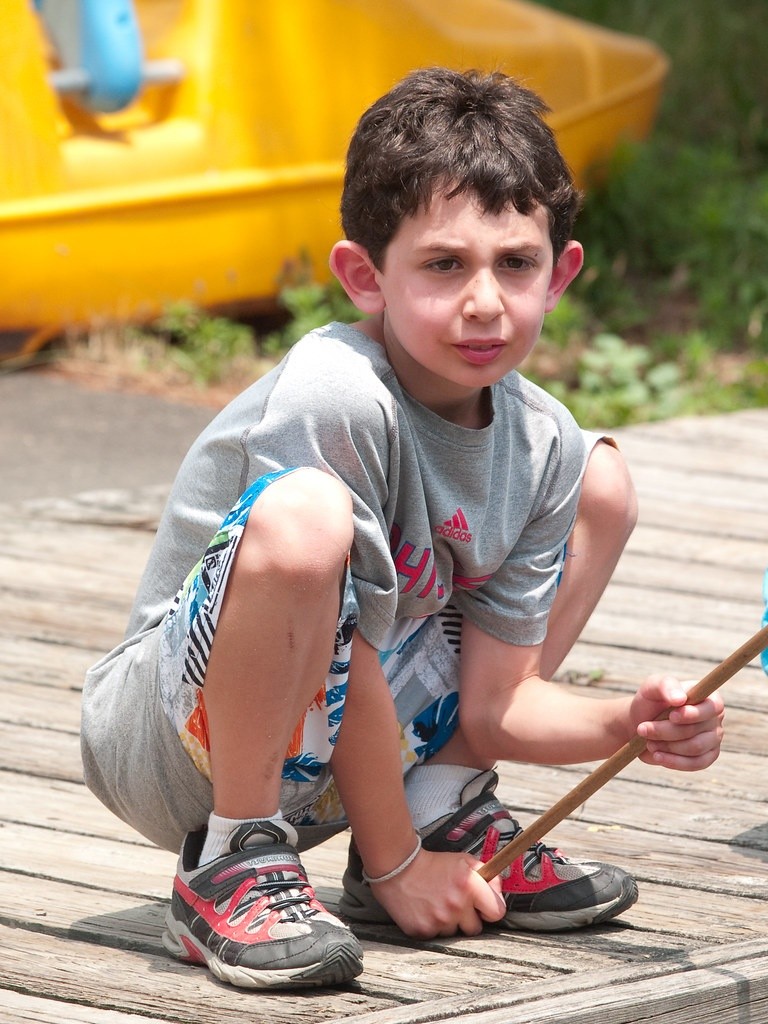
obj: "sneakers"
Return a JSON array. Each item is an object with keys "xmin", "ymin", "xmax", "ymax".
[
  {"xmin": 160, "ymin": 825, "xmax": 364, "ymax": 992},
  {"xmin": 339, "ymin": 764, "xmax": 638, "ymax": 932}
]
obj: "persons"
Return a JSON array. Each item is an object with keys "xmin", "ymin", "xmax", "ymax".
[{"xmin": 80, "ymin": 68, "xmax": 724, "ymax": 989}]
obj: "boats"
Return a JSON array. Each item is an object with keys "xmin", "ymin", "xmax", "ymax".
[{"xmin": 0, "ymin": 1, "xmax": 674, "ymax": 335}]
